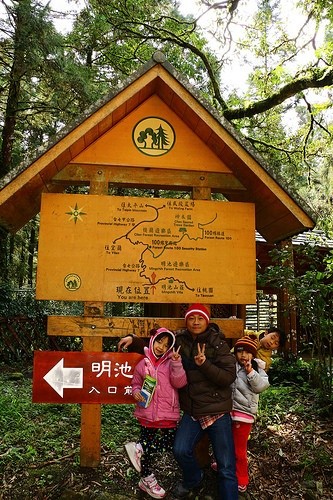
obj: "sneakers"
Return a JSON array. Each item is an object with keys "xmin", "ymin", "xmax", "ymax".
[
  {"xmin": 138, "ymin": 472, "xmax": 166, "ymax": 499},
  {"xmin": 124, "ymin": 441, "xmax": 143, "ymax": 473}
]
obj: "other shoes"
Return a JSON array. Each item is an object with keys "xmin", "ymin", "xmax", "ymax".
[
  {"xmin": 211, "ymin": 462, "xmax": 217, "ymax": 471},
  {"xmin": 175, "ymin": 470, "xmax": 204, "ymax": 497},
  {"xmin": 238, "ymin": 484, "xmax": 246, "ymax": 492}
]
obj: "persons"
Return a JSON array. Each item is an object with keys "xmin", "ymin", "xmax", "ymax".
[
  {"xmin": 117, "ymin": 304, "xmax": 237, "ymax": 500},
  {"xmin": 229, "ymin": 315, "xmax": 286, "ymax": 372},
  {"xmin": 211, "ymin": 335, "xmax": 270, "ymax": 492},
  {"xmin": 124, "ymin": 328, "xmax": 187, "ymax": 499}
]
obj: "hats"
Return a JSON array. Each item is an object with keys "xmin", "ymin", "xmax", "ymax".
[
  {"xmin": 185, "ymin": 304, "xmax": 210, "ymax": 324},
  {"xmin": 234, "ymin": 334, "xmax": 257, "ymax": 356}
]
